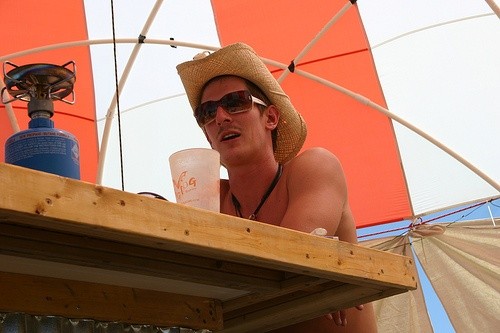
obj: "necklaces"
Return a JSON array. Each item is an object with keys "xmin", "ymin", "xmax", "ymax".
[{"xmin": 222, "ymin": 163, "xmax": 283, "ymax": 220}]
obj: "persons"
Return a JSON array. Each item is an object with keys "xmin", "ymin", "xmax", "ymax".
[{"xmin": 176, "ymin": 41, "xmax": 378, "ymax": 333}]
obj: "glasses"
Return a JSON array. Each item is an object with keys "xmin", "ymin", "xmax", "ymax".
[{"xmin": 193, "ymin": 90, "xmax": 269, "ymax": 128}]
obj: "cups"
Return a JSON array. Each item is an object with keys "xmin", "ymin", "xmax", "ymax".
[{"xmin": 169, "ymin": 148, "xmax": 221, "ymax": 213}]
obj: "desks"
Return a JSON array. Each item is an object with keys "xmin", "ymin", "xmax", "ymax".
[{"xmin": 0, "ymin": 162, "xmax": 418, "ymax": 333}]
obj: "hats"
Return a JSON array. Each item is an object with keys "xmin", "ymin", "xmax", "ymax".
[{"xmin": 176, "ymin": 42, "xmax": 307, "ymax": 163}]
